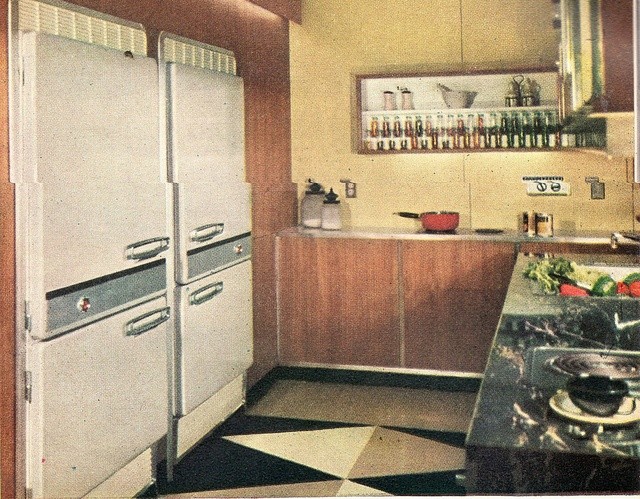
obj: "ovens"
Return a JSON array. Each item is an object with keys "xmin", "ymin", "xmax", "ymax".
[
  {"xmin": 8, "ymin": 0, "xmax": 169, "ymax": 498},
  {"xmin": 158, "ymin": 25, "xmax": 254, "ymax": 467}
]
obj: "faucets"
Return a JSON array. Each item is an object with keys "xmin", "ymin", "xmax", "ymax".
[{"xmin": 611, "ymin": 230, "xmax": 639, "ymax": 249}]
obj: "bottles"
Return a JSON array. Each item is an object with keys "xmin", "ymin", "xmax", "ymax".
[
  {"xmin": 404, "ymin": 116, "xmax": 413, "ymax": 152},
  {"xmin": 381, "ymin": 114, "xmax": 392, "ymax": 153},
  {"xmin": 468, "ymin": 113, "xmax": 477, "ymax": 148},
  {"xmin": 559, "ymin": 127, "xmax": 569, "ymax": 148},
  {"xmin": 567, "ymin": 124, "xmax": 577, "ymax": 148},
  {"xmin": 455, "ymin": 113, "xmax": 467, "ymax": 148},
  {"xmin": 546, "ymin": 111, "xmax": 557, "ymax": 148},
  {"xmin": 414, "ymin": 115, "xmax": 423, "ymax": 153},
  {"xmin": 391, "ymin": 114, "xmax": 405, "ymax": 153},
  {"xmin": 511, "ymin": 112, "xmax": 522, "ymax": 150},
  {"xmin": 424, "ymin": 115, "xmax": 435, "ymax": 150},
  {"xmin": 501, "ymin": 111, "xmax": 510, "ymax": 150},
  {"xmin": 435, "ymin": 114, "xmax": 446, "ymax": 149},
  {"xmin": 488, "ymin": 112, "xmax": 499, "ymax": 150},
  {"xmin": 477, "ymin": 113, "xmax": 488, "ymax": 148},
  {"xmin": 445, "ymin": 112, "xmax": 456, "ymax": 150},
  {"xmin": 368, "ymin": 115, "xmax": 382, "ymax": 149},
  {"xmin": 522, "ymin": 112, "xmax": 534, "ymax": 148},
  {"xmin": 535, "ymin": 111, "xmax": 546, "ymax": 149}
]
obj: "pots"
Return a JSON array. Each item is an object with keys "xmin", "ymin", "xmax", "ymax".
[{"xmin": 393, "ymin": 209, "xmax": 460, "ymax": 233}]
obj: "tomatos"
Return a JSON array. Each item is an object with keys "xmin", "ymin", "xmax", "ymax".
[
  {"xmin": 630, "ymin": 281, "xmax": 640, "ymax": 297},
  {"xmin": 615, "ymin": 281, "xmax": 629, "ymax": 296}
]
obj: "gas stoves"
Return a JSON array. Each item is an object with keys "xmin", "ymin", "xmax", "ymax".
[
  {"xmin": 414, "ymin": 227, "xmax": 518, "ymax": 239},
  {"xmin": 465, "ymin": 314, "xmax": 639, "ymax": 459}
]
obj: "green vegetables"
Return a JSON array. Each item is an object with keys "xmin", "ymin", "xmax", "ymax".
[{"xmin": 522, "ymin": 259, "xmax": 559, "ymax": 291}]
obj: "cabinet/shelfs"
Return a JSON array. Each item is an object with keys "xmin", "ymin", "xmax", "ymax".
[
  {"xmin": 274, "ymin": 227, "xmax": 640, "ymax": 382},
  {"xmin": 350, "ymin": 62, "xmax": 609, "ymax": 154},
  {"xmin": 558, "ymin": 1, "xmax": 639, "ymax": 117}
]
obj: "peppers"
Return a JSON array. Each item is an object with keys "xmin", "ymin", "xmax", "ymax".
[{"xmin": 591, "ymin": 275, "xmax": 617, "ymax": 296}]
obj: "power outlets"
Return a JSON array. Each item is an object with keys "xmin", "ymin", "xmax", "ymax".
[{"xmin": 345, "ymin": 181, "xmax": 356, "ymax": 198}]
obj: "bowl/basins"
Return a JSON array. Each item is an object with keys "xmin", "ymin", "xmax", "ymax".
[
  {"xmin": 442, "ymin": 88, "xmax": 478, "ymax": 109},
  {"xmin": 565, "ymin": 374, "xmax": 630, "ymax": 418}
]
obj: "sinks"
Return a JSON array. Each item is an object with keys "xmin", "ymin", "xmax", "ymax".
[{"xmin": 567, "ymin": 297, "xmax": 639, "ymax": 352}]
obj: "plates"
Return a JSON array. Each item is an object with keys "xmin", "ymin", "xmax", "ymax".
[{"xmin": 549, "ymin": 392, "xmax": 640, "ymax": 428}]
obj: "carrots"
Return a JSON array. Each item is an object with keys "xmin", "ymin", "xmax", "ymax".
[{"xmin": 560, "ymin": 282, "xmax": 588, "ymax": 297}]
